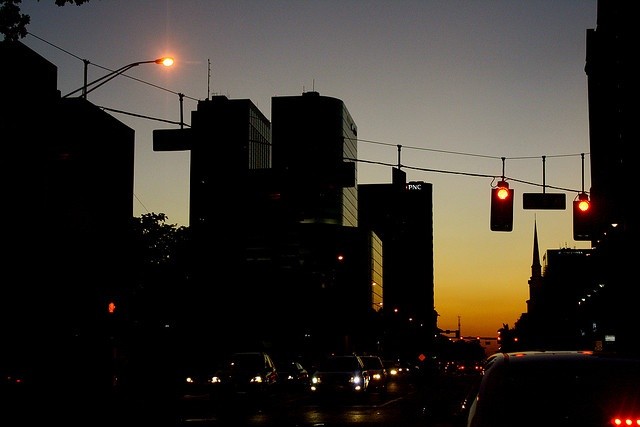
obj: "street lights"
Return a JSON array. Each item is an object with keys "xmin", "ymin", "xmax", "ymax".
[{"xmin": 61, "ymin": 58, "xmax": 174, "ymax": 99}]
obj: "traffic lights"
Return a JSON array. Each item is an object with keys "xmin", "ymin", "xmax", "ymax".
[
  {"xmin": 573, "ymin": 201, "xmax": 593, "ymax": 241},
  {"xmin": 491, "ymin": 189, "xmax": 513, "ymax": 231}
]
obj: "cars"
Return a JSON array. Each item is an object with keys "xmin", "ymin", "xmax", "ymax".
[
  {"xmin": 359, "ymin": 356, "xmax": 388, "ymax": 389},
  {"xmin": 385, "ymin": 357, "xmax": 419, "ymax": 378},
  {"xmin": 212, "ymin": 353, "xmax": 279, "ymax": 384},
  {"xmin": 467, "ymin": 351, "xmax": 611, "ymax": 427},
  {"xmin": 311, "ymin": 355, "xmax": 370, "ymax": 392}
]
